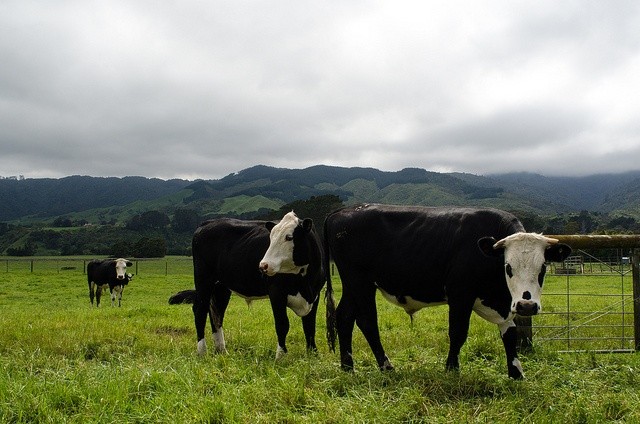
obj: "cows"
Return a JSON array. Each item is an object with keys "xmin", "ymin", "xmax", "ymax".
[
  {"xmin": 104, "ymin": 273, "xmax": 134, "ymax": 295},
  {"xmin": 322, "ymin": 203, "xmax": 573, "ymax": 380},
  {"xmin": 87, "ymin": 258, "xmax": 133, "ymax": 308},
  {"xmin": 168, "ymin": 290, "xmax": 196, "ymax": 305},
  {"xmin": 191, "ymin": 210, "xmax": 327, "ymax": 360}
]
